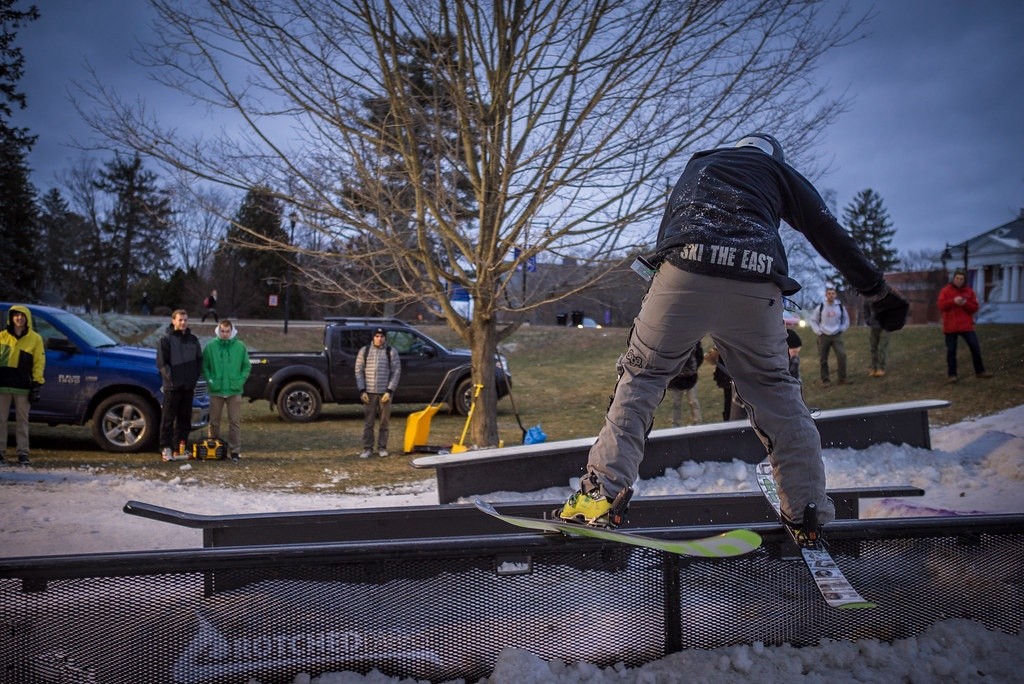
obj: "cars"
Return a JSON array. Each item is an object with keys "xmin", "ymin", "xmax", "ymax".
[
  {"xmin": 567, "ymin": 317, "xmax": 602, "ymax": 329},
  {"xmin": 783, "ymin": 309, "xmax": 806, "ymax": 327}
]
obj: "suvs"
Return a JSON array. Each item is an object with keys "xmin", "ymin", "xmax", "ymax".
[{"xmin": 0, "ymin": 301, "xmax": 212, "ymax": 454}]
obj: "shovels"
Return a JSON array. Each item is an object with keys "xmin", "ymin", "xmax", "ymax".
[
  {"xmin": 495, "ymin": 347, "xmax": 527, "ymax": 445},
  {"xmin": 450, "ymin": 385, "xmax": 485, "ymax": 454}
]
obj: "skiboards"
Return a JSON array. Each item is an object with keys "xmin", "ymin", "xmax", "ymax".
[{"xmin": 472, "ymin": 461, "xmax": 880, "ymax": 610}]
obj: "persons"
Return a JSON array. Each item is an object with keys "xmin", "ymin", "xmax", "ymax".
[
  {"xmin": 668, "ymin": 288, "xmax": 894, "ymax": 425},
  {"xmin": 556, "ymin": 132, "xmax": 915, "ymax": 547},
  {"xmin": 937, "ymin": 271, "xmax": 995, "ymax": 382},
  {"xmin": 203, "ymin": 319, "xmax": 250, "ymax": 462},
  {"xmin": 356, "ymin": 327, "xmax": 400, "ymax": 460},
  {"xmin": 0, "ymin": 306, "xmax": 46, "ymax": 467},
  {"xmin": 202, "ymin": 289, "xmax": 220, "ymax": 325},
  {"xmin": 158, "ymin": 310, "xmax": 202, "ymax": 462}
]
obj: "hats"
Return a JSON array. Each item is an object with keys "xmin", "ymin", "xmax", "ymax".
[
  {"xmin": 372, "ymin": 326, "xmax": 387, "ymax": 338},
  {"xmin": 786, "ymin": 329, "xmax": 801, "ymax": 349}
]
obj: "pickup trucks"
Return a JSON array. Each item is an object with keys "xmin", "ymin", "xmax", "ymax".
[{"xmin": 241, "ymin": 317, "xmax": 512, "ymax": 425}]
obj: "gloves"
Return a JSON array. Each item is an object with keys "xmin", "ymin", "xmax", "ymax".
[{"xmin": 870, "ymin": 289, "xmax": 908, "ymax": 331}]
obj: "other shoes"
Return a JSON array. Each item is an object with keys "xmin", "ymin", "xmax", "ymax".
[
  {"xmin": 977, "ymin": 372, "xmax": 992, "ymax": 378},
  {"xmin": 839, "ymin": 378, "xmax": 853, "ymax": 385},
  {"xmin": 949, "ymin": 376, "xmax": 958, "ymax": 383},
  {"xmin": 868, "ymin": 370, "xmax": 875, "ymax": 376},
  {"xmin": 875, "ymin": 369, "xmax": 885, "ymax": 376},
  {"xmin": 824, "ymin": 381, "xmax": 832, "ymax": 387},
  {"xmin": 19, "ymin": 455, "xmax": 31, "ymax": 464},
  {"xmin": 230, "ymin": 452, "xmax": 240, "ymax": 460}
]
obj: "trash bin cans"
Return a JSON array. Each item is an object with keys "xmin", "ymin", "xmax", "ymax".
[
  {"xmin": 556, "ymin": 313, "xmax": 568, "ymax": 326},
  {"xmin": 571, "ymin": 310, "xmax": 584, "ymax": 326}
]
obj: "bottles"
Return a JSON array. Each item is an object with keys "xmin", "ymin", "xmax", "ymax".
[{"xmin": 179, "ymin": 441, "xmax": 185, "ymax": 455}]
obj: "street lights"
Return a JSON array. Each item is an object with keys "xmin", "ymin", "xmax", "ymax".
[
  {"xmin": 230, "ymin": 251, "xmax": 248, "ymax": 317},
  {"xmin": 283, "ymin": 211, "xmax": 298, "ymax": 334},
  {"xmin": 941, "ymin": 240, "xmax": 969, "ymax": 274},
  {"xmin": 521, "ymin": 220, "xmax": 553, "ymax": 323}
]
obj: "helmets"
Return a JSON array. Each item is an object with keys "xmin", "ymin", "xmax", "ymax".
[{"xmin": 734, "ymin": 132, "xmax": 785, "ymax": 162}]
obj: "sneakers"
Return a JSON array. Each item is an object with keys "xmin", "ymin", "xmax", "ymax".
[
  {"xmin": 162, "ymin": 448, "xmax": 174, "ymax": 462},
  {"xmin": 559, "ymin": 488, "xmax": 620, "ymax": 527},
  {"xmin": 793, "ymin": 530, "xmax": 820, "ymax": 546},
  {"xmin": 379, "ymin": 449, "xmax": 388, "ymax": 457},
  {"xmin": 359, "ymin": 450, "xmax": 372, "ymax": 459}
]
